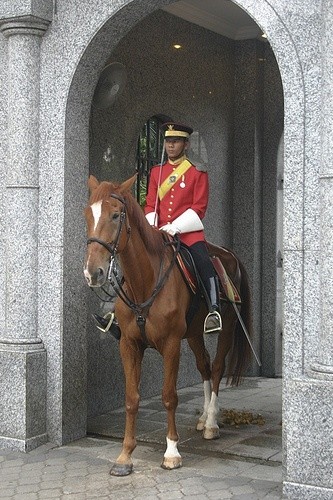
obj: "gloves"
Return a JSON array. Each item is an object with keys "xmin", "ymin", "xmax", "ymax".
[
  {"xmin": 159, "ymin": 208, "xmax": 205, "ymax": 237},
  {"xmin": 145, "ymin": 212, "xmax": 159, "ymax": 230}
]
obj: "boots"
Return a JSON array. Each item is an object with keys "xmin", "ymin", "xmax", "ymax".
[
  {"xmin": 203, "ymin": 275, "xmax": 222, "ymax": 334},
  {"xmin": 91, "ymin": 312, "xmax": 121, "ymax": 340}
]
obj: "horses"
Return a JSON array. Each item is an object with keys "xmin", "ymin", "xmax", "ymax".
[{"xmin": 83, "ymin": 172, "xmax": 253, "ymax": 478}]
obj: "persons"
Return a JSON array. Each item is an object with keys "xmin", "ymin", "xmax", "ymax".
[{"xmin": 91, "ymin": 122, "xmax": 220, "ymax": 340}]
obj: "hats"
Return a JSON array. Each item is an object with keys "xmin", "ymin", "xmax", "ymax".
[{"xmin": 162, "ymin": 123, "xmax": 193, "ymax": 142}]
